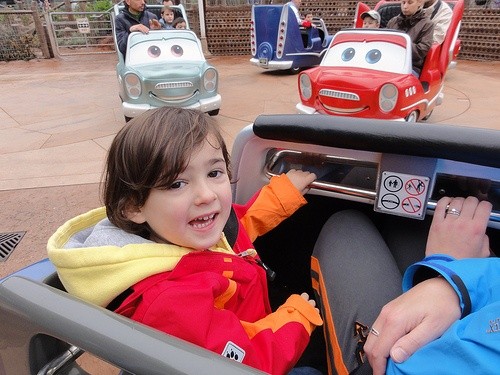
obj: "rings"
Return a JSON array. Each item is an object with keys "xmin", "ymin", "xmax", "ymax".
[
  {"xmin": 447, "ymin": 208, "xmax": 460, "ymax": 217},
  {"xmin": 371, "ymin": 328, "xmax": 380, "ymax": 336}
]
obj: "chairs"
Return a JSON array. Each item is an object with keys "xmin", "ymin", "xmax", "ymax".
[
  {"xmin": 0, "ymin": 277, "xmax": 268, "ymax": 375},
  {"xmin": 119, "ymin": 7, "xmax": 183, "ymax": 20},
  {"xmin": 299, "ymin": 20, "xmax": 325, "ymax": 48},
  {"xmin": 376, "ymin": 2, "xmax": 454, "ymax": 28}
]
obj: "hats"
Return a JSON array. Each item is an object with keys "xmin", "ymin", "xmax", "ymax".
[{"xmin": 361, "ymin": 9, "xmax": 381, "ymax": 24}]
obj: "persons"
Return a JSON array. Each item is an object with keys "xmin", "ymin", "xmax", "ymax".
[
  {"xmin": 114, "ymin": 1, "xmax": 188, "ymax": 56},
  {"xmin": 47, "ymin": 108, "xmax": 326, "ymax": 375},
  {"xmin": 361, "ymin": 1, "xmax": 453, "ymax": 93},
  {"xmin": 288, "ymin": 0, "xmax": 324, "ymax": 49},
  {"xmin": 310, "ymin": 195, "xmax": 500, "ymax": 375}
]
